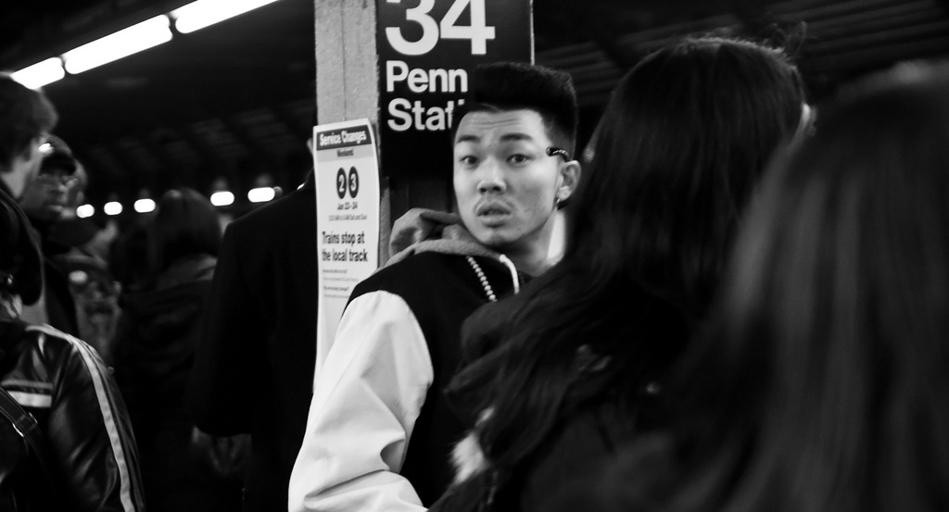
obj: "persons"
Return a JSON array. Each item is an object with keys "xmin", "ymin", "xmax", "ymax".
[
  {"xmin": 0, "ymin": 71, "xmax": 317, "ymax": 512},
  {"xmin": 537, "ymin": 62, "xmax": 946, "ymax": 511},
  {"xmin": 283, "ymin": 52, "xmax": 587, "ymax": 511},
  {"xmin": 426, "ymin": 39, "xmax": 819, "ymax": 509}
]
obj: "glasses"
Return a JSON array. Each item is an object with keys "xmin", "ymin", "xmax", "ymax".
[{"xmin": 30, "ymin": 175, "xmax": 82, "ymax": 190}]
